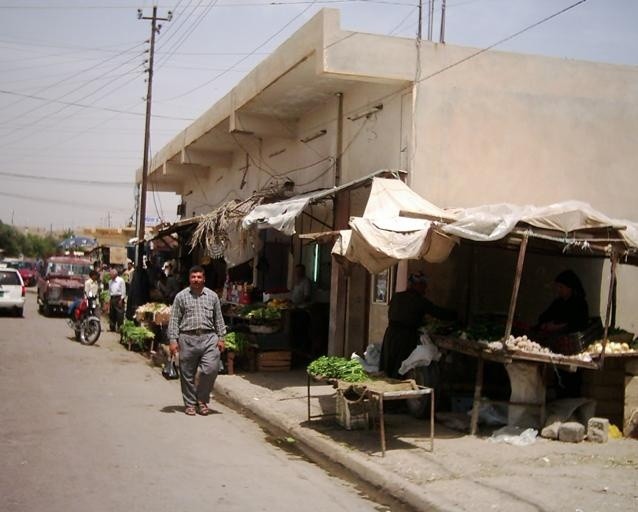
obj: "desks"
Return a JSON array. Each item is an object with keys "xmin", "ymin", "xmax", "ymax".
[{"xmin": 306, "ymin": 367, "xmax": 436, "ymax": 456}]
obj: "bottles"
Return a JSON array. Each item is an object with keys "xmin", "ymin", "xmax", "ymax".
[{"xmin": 227, "ymin": 282, "xmax": 247, "ymax": 304}]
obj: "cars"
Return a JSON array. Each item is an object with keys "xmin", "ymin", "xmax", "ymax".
[{"xmin": 0, "ymin": 256, "xmax": 92, "ymax": 317}]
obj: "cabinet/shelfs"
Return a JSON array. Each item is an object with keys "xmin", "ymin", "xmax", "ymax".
[
  {"xmin": 120, "ymin": 332, "xmax": 154, "ymax": 353},
  {"xmin": 223, "ymin": 312, "xmax": 284, "ymax": 375},
  {"xmin": 583, "ymin": 350, "xmax": 638, "ymax": 436},
  {"xmin": 430, "ymin": 333, "xmax": 600, "ymax": 435}
]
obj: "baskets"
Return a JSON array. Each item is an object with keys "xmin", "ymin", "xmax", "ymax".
[{"xmin": 257, "ymin": 350, "xmax": 293, "ymax": 372}]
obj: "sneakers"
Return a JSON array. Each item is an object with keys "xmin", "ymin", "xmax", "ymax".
[
  {"xmin": 185, "ymin": 405, "xmax": 198, "ymax": 416},
  {"xmin": 199, "ymin": 399, "xmax": 209, "ymax": 415}
]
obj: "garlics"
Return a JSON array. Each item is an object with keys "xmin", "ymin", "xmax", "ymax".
[{"xmin": 588, "ymin": 339, "xmax": 633, "ymax": 353}]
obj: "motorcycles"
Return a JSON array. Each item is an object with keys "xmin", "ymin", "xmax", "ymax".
[{"xmin": 66, "ymin": 287, "xmax": 102, "ymax": 345}]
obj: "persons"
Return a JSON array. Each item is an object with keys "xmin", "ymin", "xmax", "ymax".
[
  {"xmin": 376, "ymin": 269, "xmax": 463, "ymax": 415},
  {"xmin": 165, "ymin": 264, "xmax": 229, "ymax": 416},
  {"xmin": 535, "ymin": 269, "xmax": 589, "ymax": 422},
  {"xmin": 95, "ymin": 260, "xmax": 181, "ymax": 305},
  {"xmin": 286, "ymin": 263, "xmax": 313, "ymax": 367},
  {"xmin": 107, "ymin": 269, "xmax": 127, "ymax": 332},
  {"xmin": 85, "ymin": 269, "xmax": 99, "ymax": 316}
]
